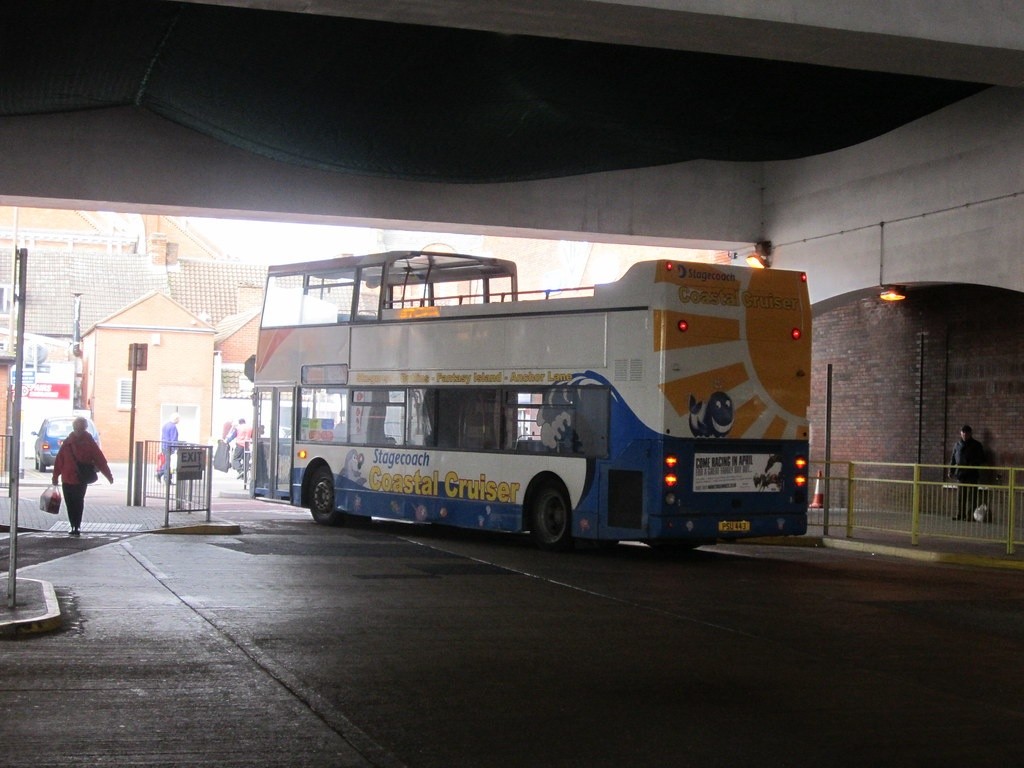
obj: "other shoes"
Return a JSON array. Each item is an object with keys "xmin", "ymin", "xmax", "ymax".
[
  {"xmin": 237, "ymin": 471, "xmax": 245, "ymax": 480},
  {"xmin": 953, "ymin": 515, "xmax": 975, "ymax": 521},
  {"xmin": 169, "ymin": 481, "xmax": 174, "ymax": 485},
  {"xmin": 155, "ymin": 473, "xmax": 161, "ymax": 483}
]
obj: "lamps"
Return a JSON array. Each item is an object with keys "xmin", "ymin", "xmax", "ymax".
[
  {"xmin": 880, "ymin": 290, "xmax": 906, "ymax": 299},
  {"xmin": 747, "ymin": 255, "xmax": 769, "ymax": 269}
]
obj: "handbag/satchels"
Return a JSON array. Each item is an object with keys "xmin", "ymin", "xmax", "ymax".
[
  {"xmin": 76, "ymin": 463, "xmax": 98, "ymax": 484},
  {"xmin": 39, "ymin": 483, "xmax": 62, "ymax": 514},
  {"xmin": 157, "ymin": 452, "xmax": 165, "ymax": 472}
]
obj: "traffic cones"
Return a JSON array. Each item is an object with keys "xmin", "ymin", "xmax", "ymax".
[{"xmin": 808, "ymin": 470, "xmax": 830, "ymax": 509}]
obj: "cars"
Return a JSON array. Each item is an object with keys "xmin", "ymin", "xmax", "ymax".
[{"xmin": 30, "ymin": 415, "xmax": 102, "ymax": 473}]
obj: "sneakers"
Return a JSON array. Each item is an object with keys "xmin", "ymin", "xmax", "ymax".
[{"xmin": 68, "ymin": 526, "xmax": 80, "ymax": 537}]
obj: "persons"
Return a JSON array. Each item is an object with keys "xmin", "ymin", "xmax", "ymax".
[
  {"xmin": 226, "ymin": 418, "xmax": 253, "ymax": 480},
  {"xmin": 948, "ymin": 426, "xmax": 989, "ymax": 521},
  {"xmin": 155, "ymin": 413, "xmax": 179, "ymax": 485},
  {"xmin": 52, "ymin": 417, "xmax": 113, "ymax": 535}
]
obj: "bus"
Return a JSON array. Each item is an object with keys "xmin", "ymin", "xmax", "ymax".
[{"xmin": 246, "ymin": 249, "xmax": 815, "ymax": 556}]
